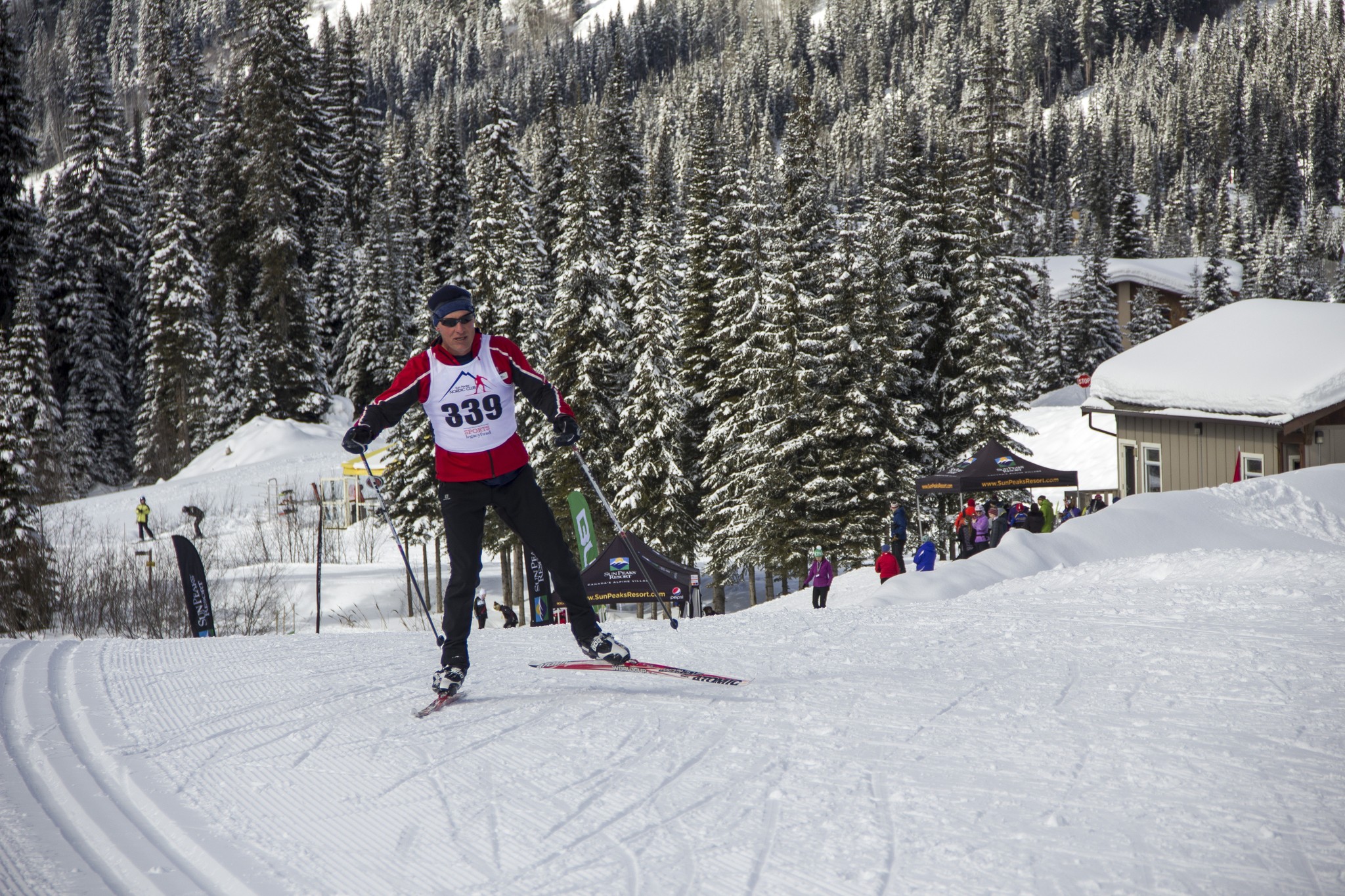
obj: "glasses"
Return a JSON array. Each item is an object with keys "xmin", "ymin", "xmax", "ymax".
[
  {"xmin": 433, "ymin": 305, "xmax": 475, "ymax": 327},
  {"xmin": 975, "ymin": 510, "xmax": 983, "ymax": 513}
]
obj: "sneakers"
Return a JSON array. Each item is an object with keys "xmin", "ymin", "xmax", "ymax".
[
  {"xmin": 580, "ymin": 633, "xmax": 631, "ymax": 665},
  {"xmin": 433, "ymin": 663, "xmax": 467, "ymax": 696}
]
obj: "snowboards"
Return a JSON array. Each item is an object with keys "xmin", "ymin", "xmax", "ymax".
[{"xmin": 171, "ymin": 534, "xmax": 216, "ymax": 640}]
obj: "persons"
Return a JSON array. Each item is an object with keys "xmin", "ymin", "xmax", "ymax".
[
  {"xmin": 494, "ymin": 601, "xmax": 519, "ymax": 628},
  {"xmin": 875, "ymin": 496, "xmax": 1121, "ymax": 585},
  {"xmin": 703, "ymin": 606, "xmax": 720, "ymax": 617},
  {"xmin": 135, "ymin": 496, "xmax": 155, "ymax": 542},
  {"xmin": 341, "ymin": 286, "xmax": 631, "ymax": 695},
  {"xmin": 182, "ymin": 505, "xmax": 205, "ymax": 539},
  {"xmin": 802, "ymin": 545, "xmax": 833, "ymax": 609},
  {"xmin": 473, "ymin": 588, "xmax": 488, "ymax": 629}
]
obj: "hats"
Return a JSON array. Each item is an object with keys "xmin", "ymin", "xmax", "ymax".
[
  {"xmin": 922, "ymin": 535, "xmax": 930, "ymax": 543},
  {"xmin": 814, "ymin": 545, "xmax": 823, "ymax": 557},
  {"xmin": 1012, "ymin": 495, "xmax": 1021, "ymax": 501},
  {"xmin": 428, "ymin": 285, "xmax": 474, "ymax": 328},
  {"xmin": 990, "ymin": 494, "xmax": 999, "ymax": 505},
  {"xmin": 494, "ymin": 602, "xmax": 500, "ymax": 611},
  {"xmin": 964, "ymin": 516, "xmax": 970, "ymax": 524},
  {"xmin": 480, "ymin": 589, "xmax": 486, "ymax": 595},
  {"xmin": 1031, "ymin": 496, "xmax": 1046, "ymax": 511},
  {"xmin": 891, "ymin": 501, "xmax": 899, "ymax": 511},
  {"xmin": 1016, "ymin": 503, "xmax": 1024, "ymax": 512},
  {"xmin": 882, "ymin": 545, "xmax": 890, "ymax": 553},
  {"xmin": 968, "ymin": 498, "xmax": 975, "ymax": 507},
  {"xmin": 1095, "ymin": 494, "xmax": 1103, "ymax": 501},
  {"xmin": 988, "ymin": 507, "xmax": 998, "ymax": 516},
  {"xmin": 975, "ymin": 505, "xmax": 984, "ymax": 512}
]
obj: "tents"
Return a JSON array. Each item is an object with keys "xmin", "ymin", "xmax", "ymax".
[
  {"xmin": 550, "ymin": 530, "xmax": 704, "ymax": 624},
  {"xmin": 342, "ymin": 440, "xmax": 408, "ymax": 526},
  {"xmin": 915, "ymin": 439, "xmax": 1079, "ymax": 545}
]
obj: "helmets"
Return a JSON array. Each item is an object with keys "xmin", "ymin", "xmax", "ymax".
[
  {"xmin": 140, "ymin": 497, "xmax": 146, "ymax": 504},
  {"xmin": 182, "ymin": 506, "xmax": 189, "ymax": 513}
]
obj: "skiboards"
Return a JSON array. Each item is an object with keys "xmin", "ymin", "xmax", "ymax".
[
  {"xmin": 131, "ymin": 537, "xmax": 156, "ymax": 545},
  {"xmin": 411, "ymin": 658, "xmax": 751, "ymax": 722}
]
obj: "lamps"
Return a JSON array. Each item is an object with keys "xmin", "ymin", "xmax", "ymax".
[
  {"xmin": 1195, "ymin": 422, "xmax": 1202, "ymax": 435},
  {"xmin": 1316, "ymin": 431, "xmax": 1324, "ymax": 444}
]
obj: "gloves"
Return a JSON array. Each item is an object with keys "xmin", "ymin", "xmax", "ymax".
[
  {"xmin": 476, "ymin": 617, "xmax": 479, "ymax": 620},
  {"xmin": 801, "ymin": 583, "xmax": 809, "ymax": 589},
  {"xmin": 486, "ymin": 616, "xmax": 488, "ymax": 618},
  {"xmin": 826, "ymin": 586, "xmax": 830, "ymax": 591},
  {"xmin": 341, "ymin": 425, "xmax": 372, "ymax": 454},
  {"xmin": 553, "ymin": 414, "xmax": 581, "ymax": 447},
  {"xmin": 890, "ymin": 535, "xmax": 899, "ymax": 543}
]
